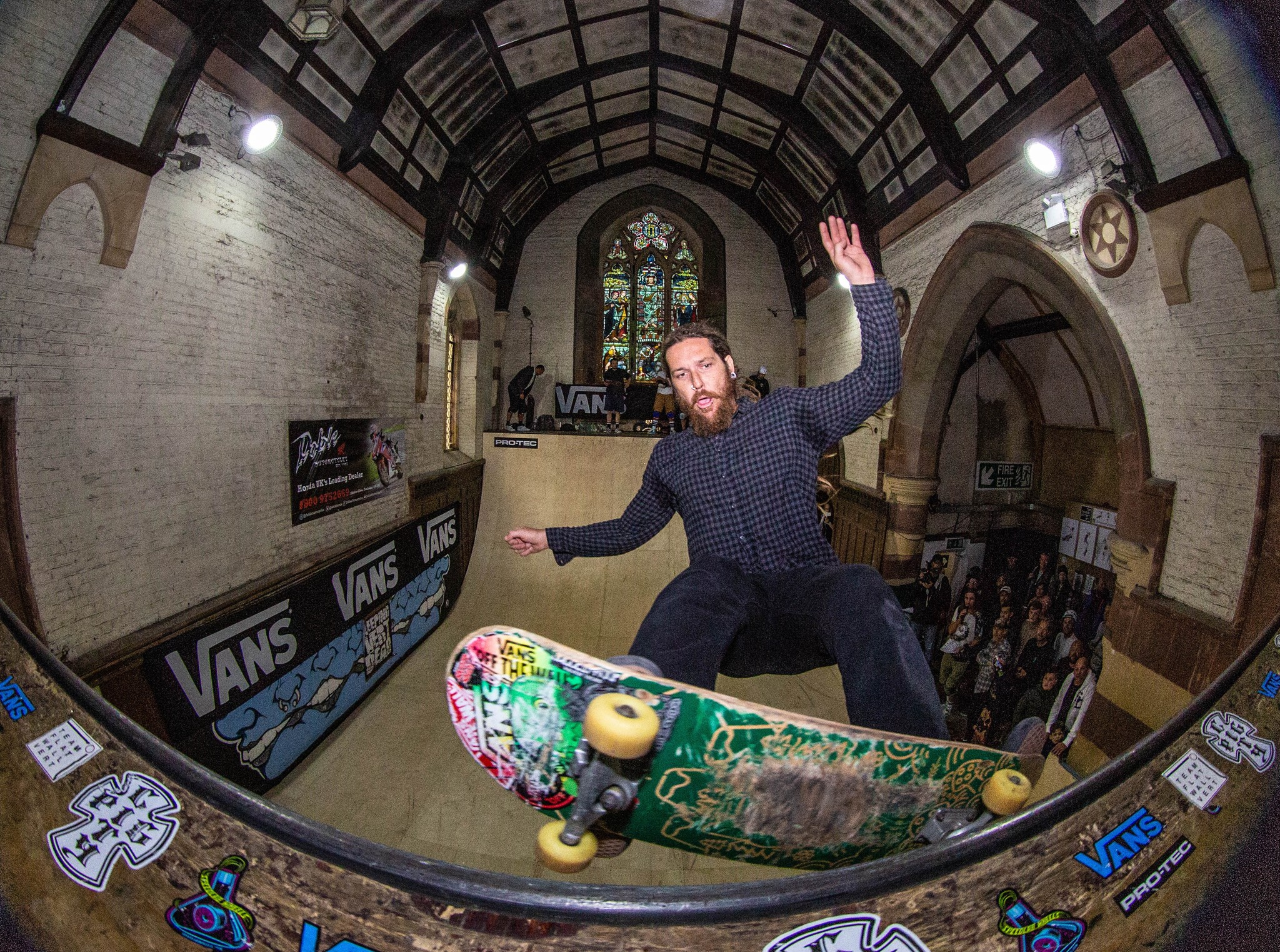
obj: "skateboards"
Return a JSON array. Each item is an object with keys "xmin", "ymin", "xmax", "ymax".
[
  {"xmin": 525, "ymin": 395, "xmax": 535, "ymax": 430},
  {"xmin": 445, "ymin": 625, "xmax": 1047, "ymax": 872}
]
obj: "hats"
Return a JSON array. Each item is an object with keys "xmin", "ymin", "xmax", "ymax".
[
  {"xmin": 930, "ymin": 554, "xmax": 943, "ymax": 562},
  {"xmin": 993, "ymin": 618, "xmax": 1008, "ymax": 629},
  {"xmin": 1000, "ymin": 586, "xmax": 1011, "ymax": 595},
  {"xmin": 968, "ymin": 563, "xmax": 983, "ymax": 577},
  {"xmin": 1062, "ymin": 610, "xmax": 1078, "ymax": 624}
]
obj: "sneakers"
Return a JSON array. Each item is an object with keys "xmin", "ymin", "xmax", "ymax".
[
  {"xmin": 670, "ymin": 430, "xmax": 677, "ymax": 435},
  {"xmin": 517, "ymin": 426, "xmax": 530, "ymax": 431},
  {"xmin": 615, "ymin": 427, "xmax": 622, "ymax": 433},
  {"xmin": 604, "ymin": 427, "xmax": 612, "ymax": 433},
  {"xmin": 504, "ymin": 425, "xmax": 515, "ymax": 431},
  {"xmin": 647, "ymin": 429, "xmax": 656, "ymax": 435}
]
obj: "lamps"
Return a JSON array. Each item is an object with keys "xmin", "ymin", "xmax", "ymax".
[
  {"xmin": 1101, "ymin": 159, "xmax": 1130, "ymax": 197},
  {"xmin": 179, "ymin": 131, "xmax": 212, "ymax": 147},
  {"xmin": 1023, "ymin": 123, "xmax": 1079, "ymax": 177},
  {"xmin": 164, "ymin": 151, "xmax": 201, "ymax": 171},
  {"xmin": 287, "ymin": 0, "xmax": 347, "ymax": 41},
  {"xmin": 768, "ymin": 308, "xmax": 793, "ymax": 317},
  {"xmin": 228, "ymin": 105, "xmax": 283, "ymax": 159}
]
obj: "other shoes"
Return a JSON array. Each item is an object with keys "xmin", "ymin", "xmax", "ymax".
[
  {"xmin": 1004, "ymin": 718, "xmax": 1046, "ymax": 755},
  {"xmin": 612, "ymin": 654, "xmax": 662, "ymax": 678}
]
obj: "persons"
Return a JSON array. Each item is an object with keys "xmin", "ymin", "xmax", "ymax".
[
  {"xmin": 503, "ymin": 216, "xmax": 1045, "ymax": 757},
  {"xmin": 502, "ymin": 357, "xmax": 770, "ymax": 435},
  {"xmin": 912, "ymin": 551, "xmax": 1112, "ymax": 756},
  {"xmin": 370, "ymin": 424, "xmax": 397, "ymax": 470}
]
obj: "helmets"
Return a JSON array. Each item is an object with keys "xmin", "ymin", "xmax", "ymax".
[{"xmin": 759, "ymin": 366, "xmax": 767, "ymax": 374}]
